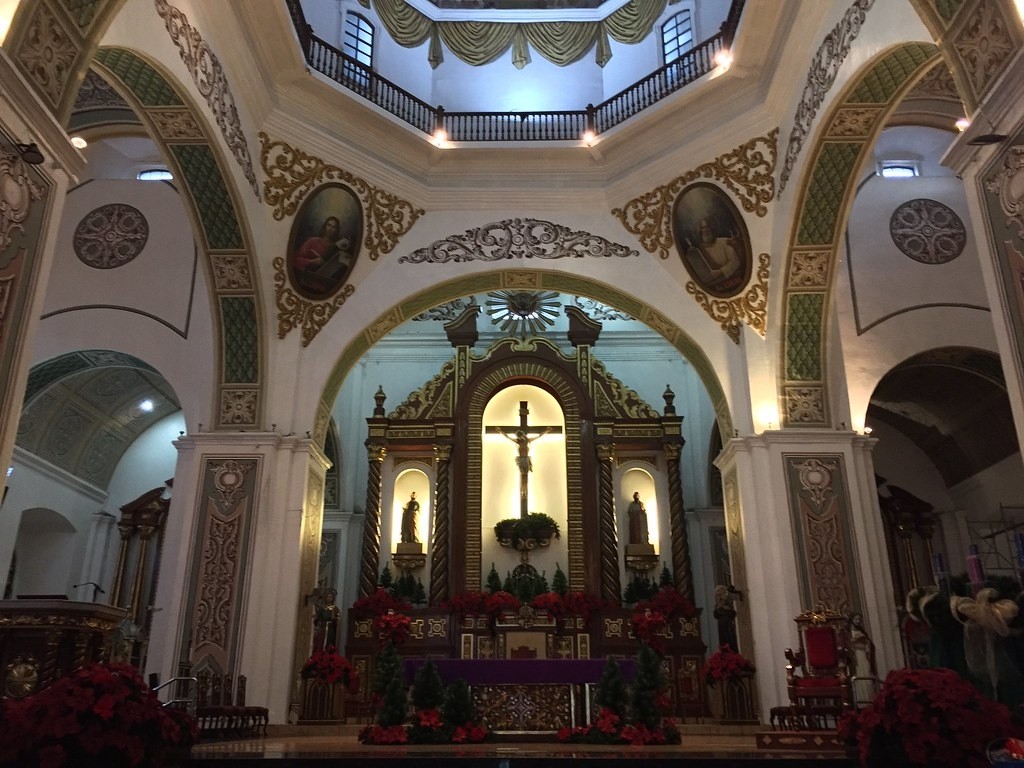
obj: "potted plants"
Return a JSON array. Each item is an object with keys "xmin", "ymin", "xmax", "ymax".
[{"xmin": 494, "ymin": 512, "xmax": 561, "ymax": 542}]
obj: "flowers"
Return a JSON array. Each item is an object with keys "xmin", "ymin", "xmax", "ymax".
[
  {"xmin": 417, "ymin": 709, "xmax": 676, "ymax": 746},
  {"xmin": 0, "ymin": 659, "xmax": 201, "ymax": 768},
  {"xmin": 299, "ymin": 645, "xmax": 362, "ymax": 695},
  {"xmin": 702, "ymin": 643, "xmax": 755, "ymax": 689},
  {"xmin": 836, "ymin": 667, "xmax": 1015, "ymax": 768},
  {"xmin": 354, "ymin": 587, "xmax": 702, "ymax": 650},
  {"xmin": 356, "ymin": 725, "xmax": 408, "ymax": 744}
]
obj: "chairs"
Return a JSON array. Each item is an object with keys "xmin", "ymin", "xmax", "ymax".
[
  {"xmin": 784, "ymin": 601, "xmax": 856, "ymax": 732},
  {"xmin": 194, "ymin": 666, "xmax": 269, "ymax": 742}
]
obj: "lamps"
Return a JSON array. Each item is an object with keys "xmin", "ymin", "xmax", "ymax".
[
  {"xmin": 662, "ymin": 385, "xmax": 675, "ymax": 406},
  {"xmin": 966, "ymin": 134, "xmax": 1007, "ymax": 146},
  {"xmin": 434, "ymin": 129, "xmax": 447, "ymax": 145},
  {"xmin": 712, "ymin": 50, "xmax": 730, "ymax": 68},
  {"xmin": 19, "ymin": 142, "xmax": 44, "ymax": 165},
  {"xmin": 583, "ymin": 130, "xmax": 595, "ymax": 147},
  {"xmin": 374, "ymin": 385, "xmax": 387, "ymax": 407}
]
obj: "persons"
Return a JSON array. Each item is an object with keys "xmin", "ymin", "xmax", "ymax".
[
  {"xmin": 628, "ymin": 493, "xmax": 649, "ymax": 544},
  {"xmin": 402, "ymin": 492, "xmax": 420, "ymax": 543},
  {"xmin": 713, "ymin": 586, "xmax": 738, "ymax": 652},
  {"xmin": 844, "ymin": 611, "xmax": 878, "ymax": 711},
  {"xmin": 314, "ymin": 592, "xmax": 340, "ymax": 652},
  {"xmin": 495, "ymin": 428, "xmax": 553, "ymax": 498}
]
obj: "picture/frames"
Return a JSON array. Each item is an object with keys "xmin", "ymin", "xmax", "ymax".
[
  {"xmin": 672, "ymin": 180, "xmax": 754, "ymax": 298},
  {"xmin": 286, "ymin": 182, "xmax": 364, "ymax": 300}
]
